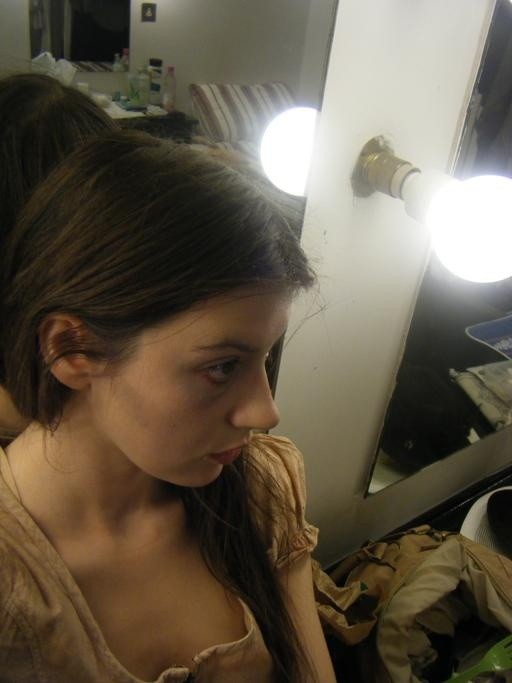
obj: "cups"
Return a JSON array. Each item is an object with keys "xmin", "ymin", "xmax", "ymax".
[{"xmin": 77, "ymin": 81, "xmax": 129, "ymax": 109}]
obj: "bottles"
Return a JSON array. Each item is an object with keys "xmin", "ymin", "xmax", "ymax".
[
  {"xmin": 162, "ymin": 65, "xmax": 178, "ymax": 108},
  {"xmin": 145, "ymin": 7, "xmax": 152, "ymax": 16},
  {"xmin": 112, "ymin": 52, "xmax": 124, "ymax": 72},
  {"xmin": 121, "ymin": 48, "xmax": 129, "ymax": 71},
  {"xmin": 128, "ymin": 65, "xmax": 150, "ymax": 102},
  {"xmin": 147, "ymin": 58, "xmax": 164, "ymax": 103}
]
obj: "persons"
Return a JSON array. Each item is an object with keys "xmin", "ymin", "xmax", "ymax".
[
  {"xmin": 1, "ymin": 129, "xmax": 341, "ymax": 683},
  {"xmin": 1, "ymin": 71, "xmax": 119, "ymax": 449}
]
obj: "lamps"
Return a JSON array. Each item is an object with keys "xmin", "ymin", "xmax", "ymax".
[{"xmin": 351, "ymin": 136, "xmax": 512, "ymax": 284}]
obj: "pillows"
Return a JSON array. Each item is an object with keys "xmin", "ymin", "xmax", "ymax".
[{"xmin": 188, "ymin": 83, "xmax": 297, "ymax": 142}]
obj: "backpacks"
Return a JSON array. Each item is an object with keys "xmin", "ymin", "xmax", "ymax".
[{"xmin": 343, "ymin": 525, "xmax": 512, "ymax": 682}]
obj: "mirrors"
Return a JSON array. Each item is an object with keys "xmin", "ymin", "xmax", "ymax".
[
  {"xmin": 366, "ymin": 0, "xmax": 512, "ymax": 496},
  {"xmin": 2, "ymin": 1, "xmax": 335, "ymax": 444}
]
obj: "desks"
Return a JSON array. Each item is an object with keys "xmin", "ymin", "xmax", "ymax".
[{"xmin": 90, "ymin": 95, "xmax": 198, "ymax": 128}]
